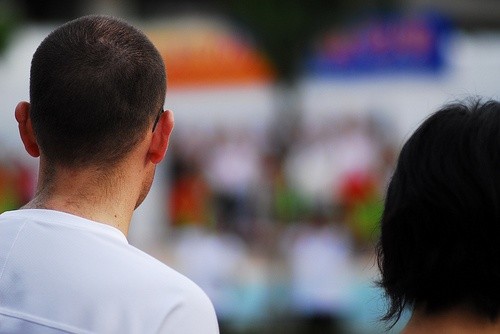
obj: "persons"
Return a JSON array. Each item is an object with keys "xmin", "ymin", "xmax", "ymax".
[
  {"xmin": 0, "ymin": 16, "xmax": 221, "ymax": 332},
  {"xmin": 376, "ymin": 99, "xmax": 500, "ymax": 334}
]
